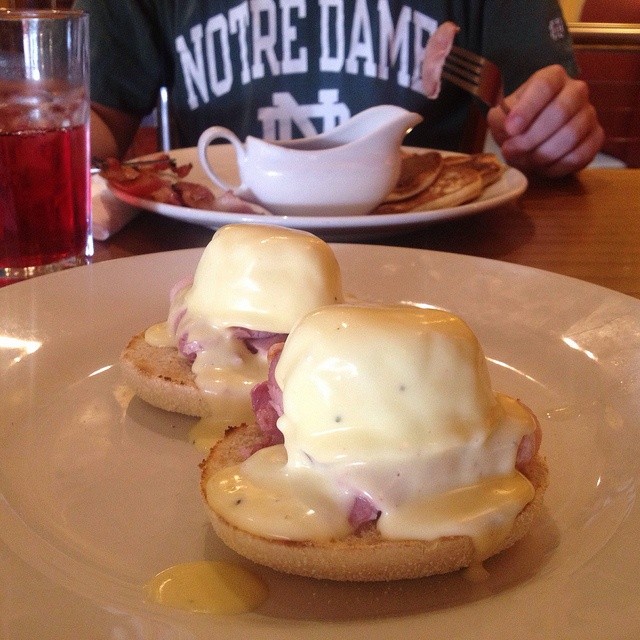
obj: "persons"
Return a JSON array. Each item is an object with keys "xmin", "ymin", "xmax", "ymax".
[{"xmin": 64, "ymin": 0, "xmax": 607, "ymax": 180}]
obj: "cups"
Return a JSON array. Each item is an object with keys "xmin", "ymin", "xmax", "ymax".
[
  {"xmin": 196, "ymin": 104, "xmax": 422, "ymax": 216},
  {"xmin": 0, "ymin": 10, "xmax": 90, "ymax": 281}
]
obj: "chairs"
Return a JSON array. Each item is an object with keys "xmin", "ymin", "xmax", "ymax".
[{"xmin": 568, "ymin": 21, "xmax": 640, "ymax": 170}]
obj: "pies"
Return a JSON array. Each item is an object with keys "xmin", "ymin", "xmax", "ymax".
[{"xmin": 378, "ymin": 148, "xmax": 506, "ymax": 213}]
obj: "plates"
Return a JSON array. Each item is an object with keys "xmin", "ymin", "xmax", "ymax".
[
  {"xmin": 106, "ymin": 140, "xmax": 529, "ymax": 234},
  {"xmin": 0, "ymin": 245, "xmax": 637, "ymax": 640}
]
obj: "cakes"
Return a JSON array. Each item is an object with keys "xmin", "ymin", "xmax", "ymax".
[
  {"xmin": 200, "ymin": 302, "xmax": 548, "ymax": 584},
  {"xmin": 119, "ymin": 223, "xmax": 357, "ymax": 419}
]
